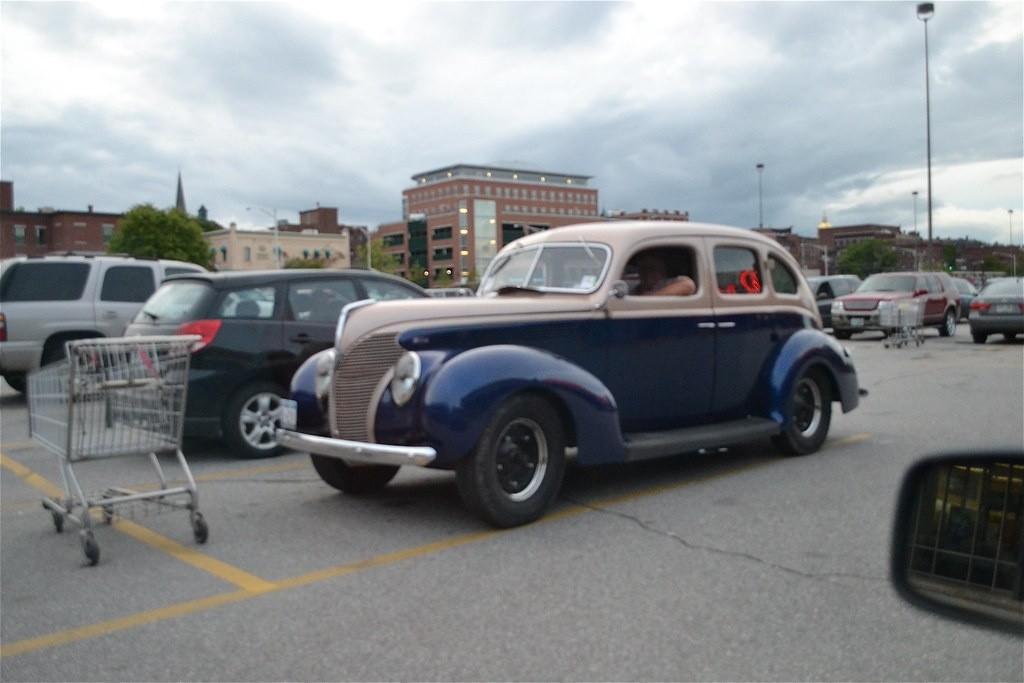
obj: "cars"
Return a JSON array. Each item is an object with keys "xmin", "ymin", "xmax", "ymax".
[
  {"xmin": 827, "ymin": 271, "xmax": 961, "ymax": 341},
  {"xmin": 113, "ymin": 269, "xmax": 446, "ymax": 459},
  {"xmin": 797, "ymin": 274, "xmax": 865, "ymax": 329},
  {"xmin": 967, "ymin": 275, "xmax": 1024, "ymax": 344},
  {"xmin": 276, "ymin": 218, "xmax": 866, "ymax": 532},
  {"xmin": 950, "ymin": 275, "xmax": 977, "ymax": 319}
]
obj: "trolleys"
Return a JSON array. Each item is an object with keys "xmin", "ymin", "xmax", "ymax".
[{"xmin": 25, "ymin": 336, "xmax": 213, "ymax": 564}]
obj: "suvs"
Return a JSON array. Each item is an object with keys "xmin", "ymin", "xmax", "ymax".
[{"xmin": 0, "ymin": 255, "xmax": 210, "ymax": 396}]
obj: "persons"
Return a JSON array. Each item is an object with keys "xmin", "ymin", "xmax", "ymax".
[{"xmin": 626, "ymin": 254, "xmax": 697, "ymax": 296}]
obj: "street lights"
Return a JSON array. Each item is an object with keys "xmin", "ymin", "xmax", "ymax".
[
  {"xmin": 915, "ymin": 2, "xmax": 938, "ymax": 243},
  {"xmin": 755, "ymin": 162, "xmax": 765, "ymax": 230}
]
